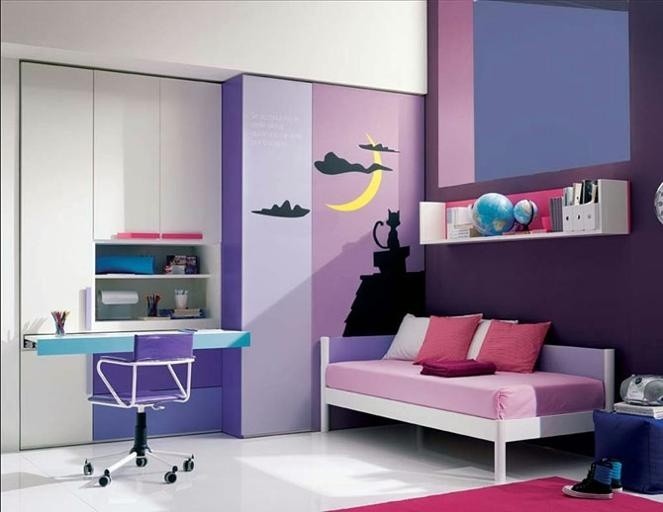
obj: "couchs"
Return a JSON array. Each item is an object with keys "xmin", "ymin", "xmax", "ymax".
[{"xmin": 319, "ymin": 326, "xmax": 615, "ymax": 482}]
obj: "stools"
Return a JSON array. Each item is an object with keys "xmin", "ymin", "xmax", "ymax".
[{"xmin": 592, "ymin": 405, "xmax": 662, "ymax": 495}]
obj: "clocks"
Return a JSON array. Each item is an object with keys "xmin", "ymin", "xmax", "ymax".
[{"xmin": 652, "ymin": 182, "xmax": 662, "ymax": 225}]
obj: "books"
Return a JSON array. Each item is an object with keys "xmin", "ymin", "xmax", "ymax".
[
  {"xmin": 547, "ymin": 178, "xmax": 600, "ymax": 232},
  {"xmin": 445, "ymin": 206, "xmax": 479, "ymax": 241},
  {"xmin": 136, "ymin": 307, "xmax": 206, "ymax": 321}
]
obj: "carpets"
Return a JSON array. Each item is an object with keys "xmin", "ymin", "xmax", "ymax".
[{"xmin": 327, "ymin": 475, "xmax": 662, "ymax": 511}]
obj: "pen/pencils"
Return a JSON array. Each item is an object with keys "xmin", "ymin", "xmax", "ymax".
[
  {"xmin": 51, "ymin": 310, "xmax": 70, "ymax": 336},
  {"xmin": 174, "ymin": 288, "xmax": 189, "ymax": 295},
  {"xmin": 146, "ymin": 294, "xmax": 161, "ymax": 317}
]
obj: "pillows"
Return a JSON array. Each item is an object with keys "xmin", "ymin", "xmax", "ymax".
[{"xmin": 377, "ymin": 312, "xmax": 552, "ymax": 375}]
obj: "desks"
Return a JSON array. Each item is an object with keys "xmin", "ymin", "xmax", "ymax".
[{"xmin": 23, "ymin": 327, "xmax": 251, "ymax": 359}]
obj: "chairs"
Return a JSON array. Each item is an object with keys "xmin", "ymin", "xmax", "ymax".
[{"xmin": 80, "ymin": 334, "xmax": 199, "ymax": 488}]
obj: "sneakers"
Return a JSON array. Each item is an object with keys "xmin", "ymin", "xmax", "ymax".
[{"xmin": 563, "ymin": 460, "xmax": 625, "ymax": 497}]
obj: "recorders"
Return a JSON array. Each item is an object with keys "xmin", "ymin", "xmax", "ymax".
[{"xmin": 620, "ymin": 374, "xmax": 663, "ymax": 406}]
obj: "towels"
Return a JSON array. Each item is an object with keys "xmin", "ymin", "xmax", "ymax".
[{"xmin": 421, "ymin": 358, "xmax": 495, "ymax": 378}]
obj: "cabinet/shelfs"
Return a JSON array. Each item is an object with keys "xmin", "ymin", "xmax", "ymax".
[
  {"xmin": 92, "ymin": 69, "xmax": 222, "ymax": 248},
  {"xmin": 93, "ymin": 238, "xmax": 214, "ymax": 327},
  {"xmin": 20, "ymin": 60, "xmax": 93, "ymax": 337}
]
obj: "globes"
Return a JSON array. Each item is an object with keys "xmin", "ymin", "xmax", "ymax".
[
  {"xmin": 514, "ymin": 199, "xmax": 538, "ymax": 234},
  {"xmin": 472, "ymin": 192, "xmax": 520, "ymax": 236}
]
